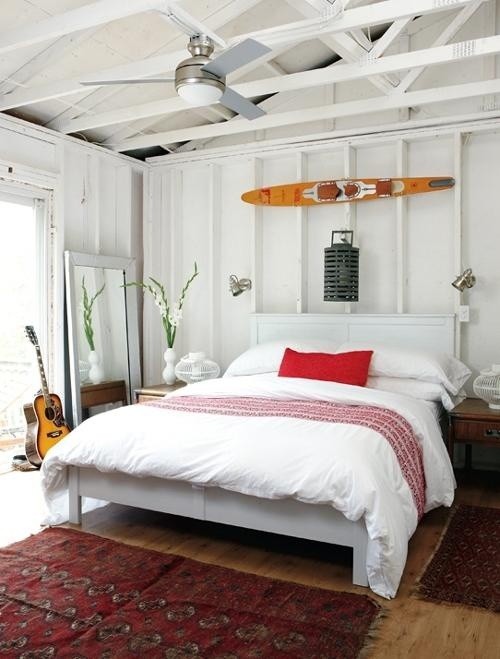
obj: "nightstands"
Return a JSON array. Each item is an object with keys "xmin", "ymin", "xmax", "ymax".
[
  {"xmin": 133, "ymin": 381, "xmax": 187, "ymax": 403},
  {"xmin": 447, "ymin": 398, "xmax": 500, "ymax": 472},
  {"xmin": 81, "ymin": 381, "xmax": 128, "ymax": 421}
]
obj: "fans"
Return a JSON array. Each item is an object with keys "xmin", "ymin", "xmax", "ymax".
[{"xmin": 79, "ymin": 31, "xmax": 268, "ymax": 121}]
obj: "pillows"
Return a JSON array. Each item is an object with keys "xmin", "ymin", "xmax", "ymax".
[{"xmin": 222, "ymin": 339, "xmax": 472, "ymax": 413}]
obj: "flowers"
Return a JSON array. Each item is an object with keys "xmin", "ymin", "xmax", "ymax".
[
  {"xmin": 77, "ymin": 275, "xmax": 107, "ymax": 351},
  {"xmin": 118, "ymin": 262, "xmax": 201, "ymax": 348}
]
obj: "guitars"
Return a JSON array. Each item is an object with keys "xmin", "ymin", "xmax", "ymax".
[{"xmin": 23, "ymin": 323, "xmax": 71, "ymax": 467}]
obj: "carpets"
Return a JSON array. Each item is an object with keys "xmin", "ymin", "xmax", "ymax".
[
  {"xmin": 0, "ymin": 526, "xmax": 390, "ymax": 659},
  {"xmin": 409, "ymin": 503, "xmax": 500, "ymax": 613}
]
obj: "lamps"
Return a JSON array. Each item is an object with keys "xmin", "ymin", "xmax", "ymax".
[
  {"xmin": 323, "ymin": 230, "xmax": 359, "ymax": 302},
  {"xmin": 175, "ymin": 352, "xmax": 220, "ymax": 385},
  {"xmin": 228, "ymin": 274, "xmax": 251, "ymax": 296},
  {"xmin": 175, "ymin": 82, "xmax": 223, "ymax": 108},
  {"xmin": 473, "ymin": 364, "xmax": 500, "ymax": 410},
  {"xmin": 452, "ymin": 268, "xmax": 476, "ymax": 294}
]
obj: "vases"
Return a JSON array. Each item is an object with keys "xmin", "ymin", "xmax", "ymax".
[
  {"xmin": 88, "ymin": 349, "xmax": 103, "ymax": 386},
  {"xmin": 162, "ymin": 349, "xmax": 177, "ymax": 385}
]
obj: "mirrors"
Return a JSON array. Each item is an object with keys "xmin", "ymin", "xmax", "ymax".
[{"xmin": 64, "ymin": 250, "xmax": 142, "ymax": 430}]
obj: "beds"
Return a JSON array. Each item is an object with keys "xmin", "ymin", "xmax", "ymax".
[{"xmin": 39, "ymin": 313, "xmax": 456, "ymax": 599}]
